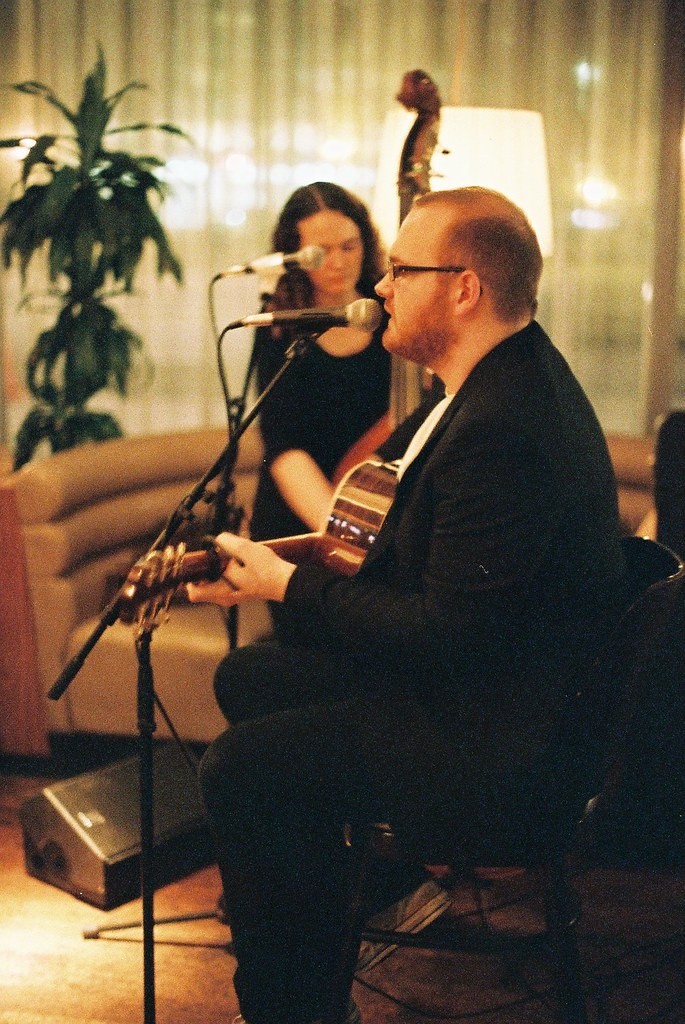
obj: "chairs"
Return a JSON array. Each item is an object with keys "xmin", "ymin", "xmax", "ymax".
[{"xmin": 329, "ymin": 534, "xmax": 685, "ymax": 1024}]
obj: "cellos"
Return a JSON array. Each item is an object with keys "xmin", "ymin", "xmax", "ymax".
[{"xmin": 328, "ymin": 68, "xmax": 443, "ymax": 489}]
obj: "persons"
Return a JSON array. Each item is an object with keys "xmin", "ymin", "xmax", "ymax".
[
  {"xmin": 184, "ymin": 188, "xmax": 636, "ymax": 1024},
  {"xmin": 247, "ymin": 182, "xmax": 432, "ymax": 631}
]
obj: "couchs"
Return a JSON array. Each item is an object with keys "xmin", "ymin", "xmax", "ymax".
[{"xmin": 0, "ymin": 428, "xmax": 656, "ymax": 740}]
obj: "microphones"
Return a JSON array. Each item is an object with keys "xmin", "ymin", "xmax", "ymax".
[
  {"xmin": 219, "ymin": 246, "xmax": 324, "ymax": 278},
  {"xmin": 229, "ymin": 297, "xmax": 383, "ymax": 332}
]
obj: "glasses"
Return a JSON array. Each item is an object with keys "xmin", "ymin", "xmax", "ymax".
[{"xmin": 387, "ymin": 261, "xmax": 483, "ymax": 297}]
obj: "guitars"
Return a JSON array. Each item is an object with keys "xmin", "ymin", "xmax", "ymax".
[{"xmin": 120, "ymin": 459, "xmax": 400, "ymax": 641}]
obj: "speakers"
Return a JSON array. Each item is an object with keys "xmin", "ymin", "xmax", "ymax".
[{"xmin": 18, "ymin": 744, "xmax": 218, "ymax": 911}]
